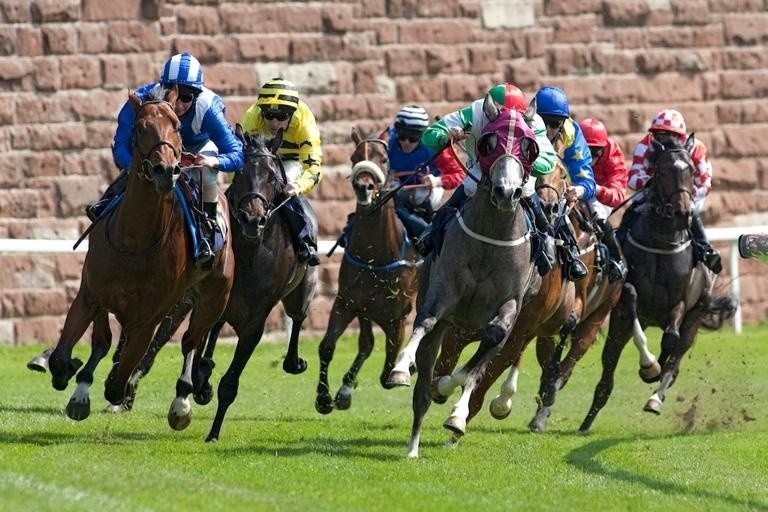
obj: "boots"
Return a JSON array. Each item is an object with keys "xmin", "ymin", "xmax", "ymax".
[
  {"xmin": 269, "ymin": 188, "xmax": 321, "ymax": 268},
  {"xmin": 689, "ymin": 211, "xmax": 723, "ymax": 274},
  {"xmin": 522, "ymin": 192, "xmax": 557, "ymax": 265},
  {"xmin": 556, "ymin": 221, "xmax": 587, "ymax": 278},
  {"xmin": 593, "ymin": 217, "xmax": 628, "ymax": 280},
  {"xmin": 413, "ymin": 183, "xmax": 471, "ymax": 258},
  {"xmin": 85, "ymin": 167, "xmax": 131, "ymax": 224}
]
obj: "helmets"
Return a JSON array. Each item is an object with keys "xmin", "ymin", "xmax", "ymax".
[
  {"xmin": 161, "ymin": 52, "xmax": 205, "ymax": 92},
  {"xmin": 396, "ymin": 104, "xmax": 430, "ymax": 130},
  {"xmin": 483, "ymin": 82, "xmax": 528, "ymax": 122},
  {"xmin": 579, "ymin": 117, "xmax": 609, "ymax": 147},
  {"xmin": 533, "ymin": 85, "xmax": 570, "ymax": 119},
  {"xmin": 649, "ymin": 109, "xmax": 687, "ymax": 137},
  {"xmin": 256, "ymin": 78, "xmax": 299, "ymax": 109}
]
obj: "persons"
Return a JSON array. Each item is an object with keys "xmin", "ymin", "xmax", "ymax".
[
  {"xmin": 337, "ymin": 105, "xmax": 464, "ymax": 257},
  {"xmin": 86, "ymin": 52, "xmax": 245, "ymax": 270},
  {"xmin": 606, "ymin": 109, "xmax": 721, "ymax": 283},
  {"xmin": 529, "ymin": 86, "xmax": 595, "ymax": 279},
  {"xmin": 415, "ymin": 83, "xmax": 556, "ymax": 276},
  {"xmin": 224, "ymin": 78, "xmax": 323, "ymax": 265},
  {"xmin": 581, "ymin": 118, "xmax": 629, "ymax": 283}
]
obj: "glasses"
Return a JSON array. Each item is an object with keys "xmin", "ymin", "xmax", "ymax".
[
  {"xmin": 592, "ymin": 148, "xmax": 600, "ymax": 159},
  {"xmin": 540, "ymin": 120, "xmax": 561, "ymax": 129},
  {"xmin": 165, "ymin": 93, "xmax": 194, "ymax": 103},
  {"xmin": 261, "ymin": 112, "xmax": 293, "ymax": 122},
  {"xmin": 396, "ymin": 133, "xmax": 420, "ymax": 143},
  {"xmin": 654, "ymin": 133, "xmax": 674, "ymax": 140}
]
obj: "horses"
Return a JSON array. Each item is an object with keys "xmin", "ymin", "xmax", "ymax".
[
  {"xmin": 25, "ymin": 82, "xmax": 320, "ymax": 433},
  {"xmin": 578, "ymin": 131, "xmax": 737, "ymax": 431},
  {"xmin": 47, "ymin": 83, "xmax": 236, "ymax": 433},
  {"xmin": 315, "ymin": 122, "xmax": 626, "ymax": 435},
  {"xmin": 386, "ymin": 93, "xmax": 569, "ymax": 461},
  {"xmin": 26, "ymin": 120, "xmax": 314, "ymax": 444}
]
